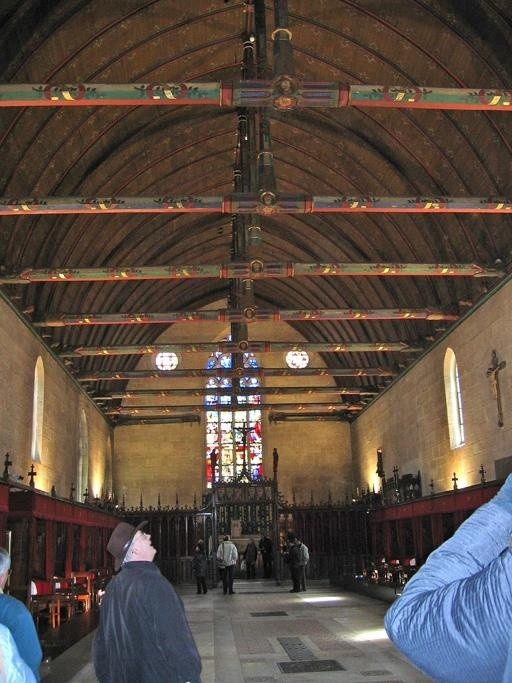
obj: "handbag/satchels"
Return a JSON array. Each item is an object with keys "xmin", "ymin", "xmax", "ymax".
[{"xmin": 216, "ymin": 558, "xmax": 225, "ymax": 569}]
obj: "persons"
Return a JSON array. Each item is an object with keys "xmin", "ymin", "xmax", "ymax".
[
  {"xmin": 216, "ymin": 535, "xmax": 238, "ymax": 595},
  {"xmin": 289, "ymin": 538, "xmax": 309, "ymax": 592},
  {"xmin": 243, "ymin": 538, "xmax": 257, "ymax": 579},
  {"xmin": 384, "ymin": 469, "xmax": 512, "ymax": 683},
  {"xmin": 192, "ymin": 544, "xmax": 207, "ymax": 594},
  {"xmin": 93, "ymin": 521, "xmax": 201, "ymax": 683},
  {"xmin": 0, "ymin": 546, "xmax": 43, "ymax": 683},
  {"xmin": 259, "ymin": 533, "xmax": 273, "ymax": 577}
]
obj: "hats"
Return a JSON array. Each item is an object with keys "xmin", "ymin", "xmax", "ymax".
[{"xmin": 107, "ymin": 520, "xmax": 149, "ymax": 572}]
